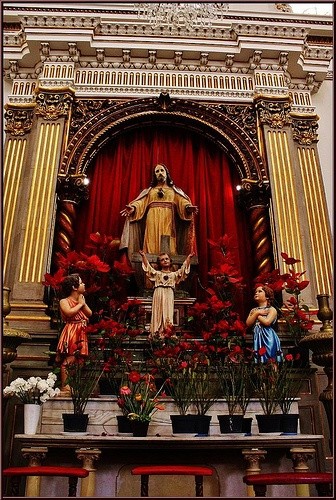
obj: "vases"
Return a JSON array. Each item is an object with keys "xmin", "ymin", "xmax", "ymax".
[
  {"xmin": 116, "ymin": 416, "xmax": 150, "ymax": 437},
  {"xmin": 170, "ymin": 415, "xmax": 212, "ymax": 435},
  {"xmin": 243, "ymin": 418, "xmax": 254, "ymax": 433},
  {"xmin": 62, "ymin": 413, "xmax": 89, "ymax": 433},
  {"xmin": 256, "ymin": 414, "xmax": 300, "ymax": 434},
  {"xmin": 217, "ymin": 415, "xmax": 243, "ymax": 432},
  {"xmin": 24, "ymin": 404, "xmax": 41, "ymax": 434}
]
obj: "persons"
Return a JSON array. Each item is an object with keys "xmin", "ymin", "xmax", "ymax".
[
  {"xmin": 138, "ymin": 250, "xmax": 195, "ymax": 339},
  {"xmin": 246, "ymin": 284, "xmax": 286, "ymax": 391},
  {"xmin": 56, "ymin": 274, "xmax": 92, "ymax": 392},
  {"xmin": 120, "ymin": 162, "xmax": 199, "ymax": 255}
]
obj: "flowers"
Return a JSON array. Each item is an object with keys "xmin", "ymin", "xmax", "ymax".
[{"xmin": 3, "ymin": 230, "xmax": 319, "ymax": 420}]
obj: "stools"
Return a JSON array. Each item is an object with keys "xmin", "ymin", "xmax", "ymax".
[
  {"xmin": 3, "ymin": 467, "xmax": 89, "ymax": 497},
  {"xmin": 243, "ymin": 471, "xmax": 333, "ymax": 497},
  {"xmin": 131, "ymin": 465, "xmax": 212, "ymax": 497}
]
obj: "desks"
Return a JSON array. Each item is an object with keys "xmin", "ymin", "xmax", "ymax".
[{"xmin": 126, "ymin": 297, "xmax": 196, "ymax": 332}]
